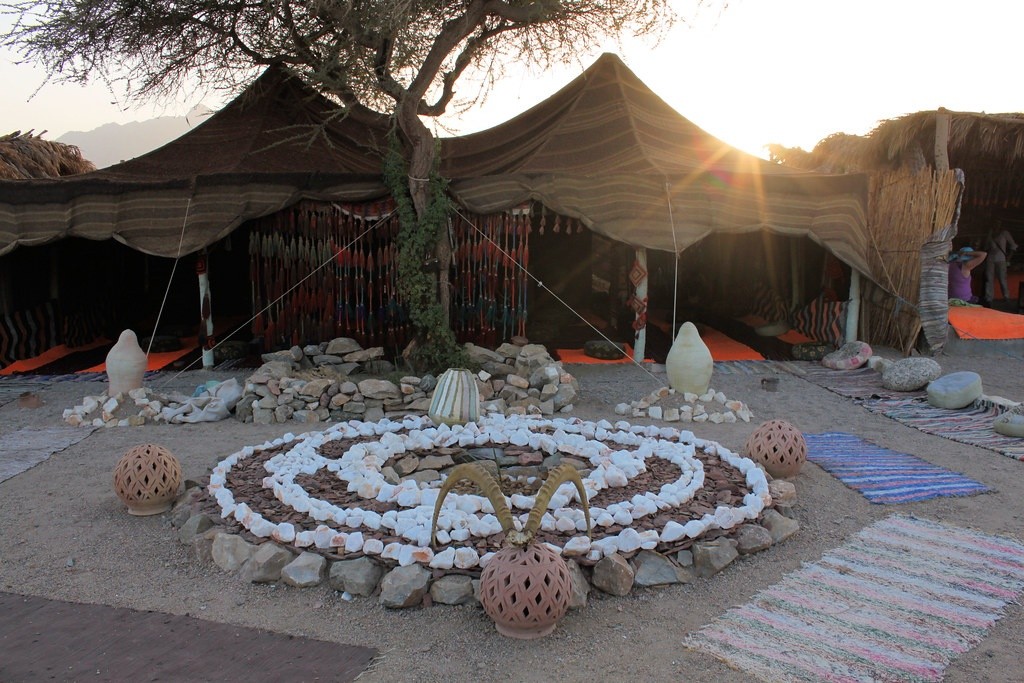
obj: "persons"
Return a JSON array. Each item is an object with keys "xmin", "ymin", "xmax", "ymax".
[
  {"xmin": 950, "ymin": 246, "xmax": 987, "ymax": 304},
  {"xmin": 983, "ymin": 218, "xmax": 1018, "ymax": 309}
]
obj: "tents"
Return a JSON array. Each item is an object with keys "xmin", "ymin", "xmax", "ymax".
[
  {"xmin": 434, "ymin": 52, "xmax": 881, "ymax": 365},
  {"xmin": 761, "ymin": 108, "xmax": 1024, "ymax": 357},
  {"xmin": 1, "ymin": 58, "xmax": 413, "ymax": 375}
]
